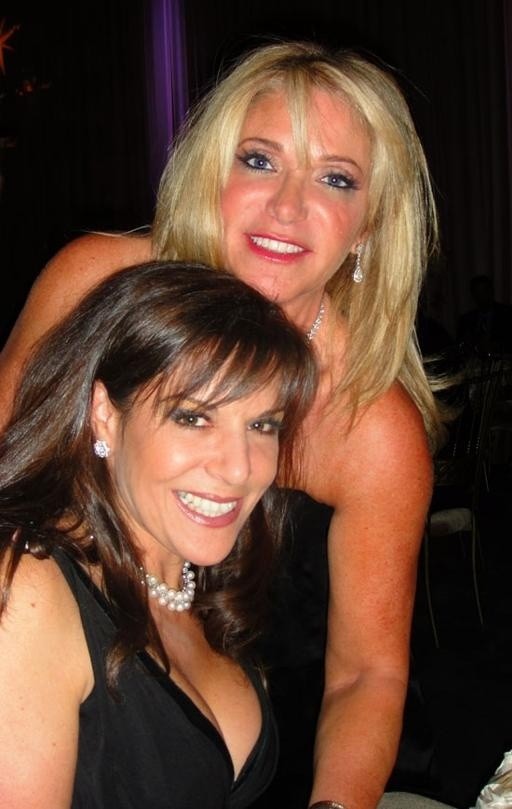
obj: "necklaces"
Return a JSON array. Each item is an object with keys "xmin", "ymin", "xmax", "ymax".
[
  {"xmin": 306, "ymin": 299, "xmax": 325, "ymax": 343},
  {"xmin": 139, "ymin": 560, "xmax": 197, "ymax": 613}
]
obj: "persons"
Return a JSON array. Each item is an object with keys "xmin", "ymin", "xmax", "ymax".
[
  {"xmin": 0, "ymin": 38, "xmax": 450, "ymax": 809},
  {"xmin": 2, "ymin": 260, "xmax": 323, "ymax": 809}
]
